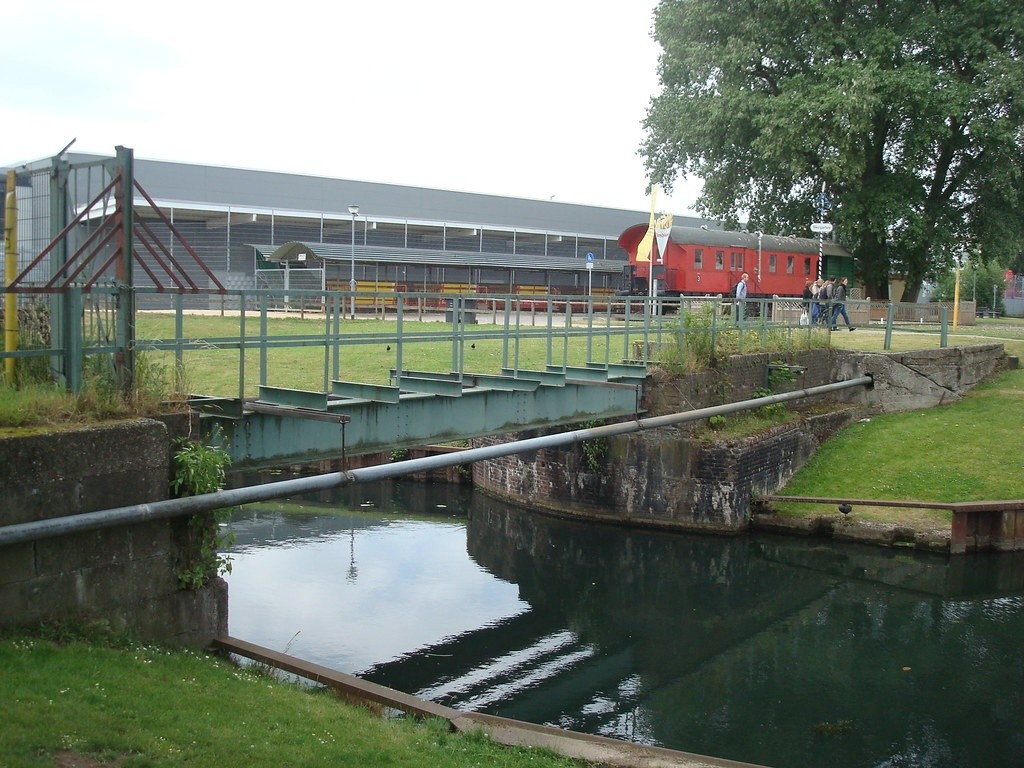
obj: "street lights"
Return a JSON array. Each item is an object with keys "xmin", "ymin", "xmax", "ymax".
[{"xmin": 348, "ymin": 203, "xmax": 360, "ymax": 321}]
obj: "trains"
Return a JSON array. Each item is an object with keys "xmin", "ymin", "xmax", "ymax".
[{"xmin": 615, "ymin": 222, "xmax": 821, "ymax": 311}]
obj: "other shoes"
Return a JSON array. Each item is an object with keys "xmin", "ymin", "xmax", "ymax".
[
  {"xmin": 849, "ymin": 327, "xmax": 856, "ymax": 332},
  {"xmin": 831, "ymin": 326, "xmax": 840, "ymax": 331}
]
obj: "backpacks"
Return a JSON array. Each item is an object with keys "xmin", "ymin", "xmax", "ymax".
[
  {"xmin": 732, "ymin": 281, "xmax": 744, "ymax": 298},
  {"xmin": 820, "ymin": 287, "xmax": 827, "ymax": 299}
]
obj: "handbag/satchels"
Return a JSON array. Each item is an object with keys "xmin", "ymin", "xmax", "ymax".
[{"xmin": 800, "ymin": 311, "xmax": 809, "ymax": 325}]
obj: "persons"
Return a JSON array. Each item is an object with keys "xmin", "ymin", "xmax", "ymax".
[
  {"xmin": 803, "ymin": 275, "xmax": 856, "ymax": 332},
  {"xmin": 731, "ymin": 273, "xmax": 749, "ymax": 328}
]
